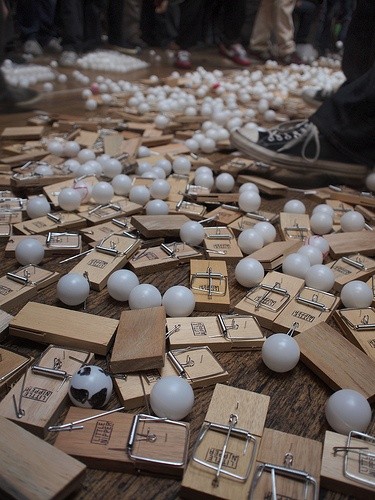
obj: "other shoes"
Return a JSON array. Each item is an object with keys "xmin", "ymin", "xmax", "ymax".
[
  {"xmin": 113, "ymin": 41, "xmax": 142, "ymax": 53},
  {"xmin": 173, "ymin": 49, "xmax": 194, "ymax": 69},
  {"xmin": 299, "ymin": 44, "xmax": 319, "ymax": 62},
  {"xmin": 281, "ymin": 54, "xmax": 303, "ymax": 64},
  {"xmin": 49, "ymin": 37, "xmax": 63, "ymax": 52},
  {"xmin": 25, "ymin": 41, "xmax": 43, "ymax": 56},
  {"xmin": 228, "ymin": 44, "xmax": 254, "ymax": 66}
]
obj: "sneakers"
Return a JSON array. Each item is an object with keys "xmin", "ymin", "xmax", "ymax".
[
  {"xmin": 7, "ymin": 86, "xmax": 43, "ymax": 108},
  {"xmin": 299, "ymin": 85, "xmax": 341, "ymax": 104},
  {"xmin": 229, "ymin": 117, "xmax": 370, "ymax": 178}
]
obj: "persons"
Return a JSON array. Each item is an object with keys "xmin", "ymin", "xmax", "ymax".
[
  {"xmin": 0, "ymin": 0, "xmax": 251, "ymax": 106},
  {"xmin": 230, "ymin": 0, "xmax": 375, "ymax": 179},
  {"xmin": 247, "ymin": 0, "xmax": 356, "ymax": 64}
]
